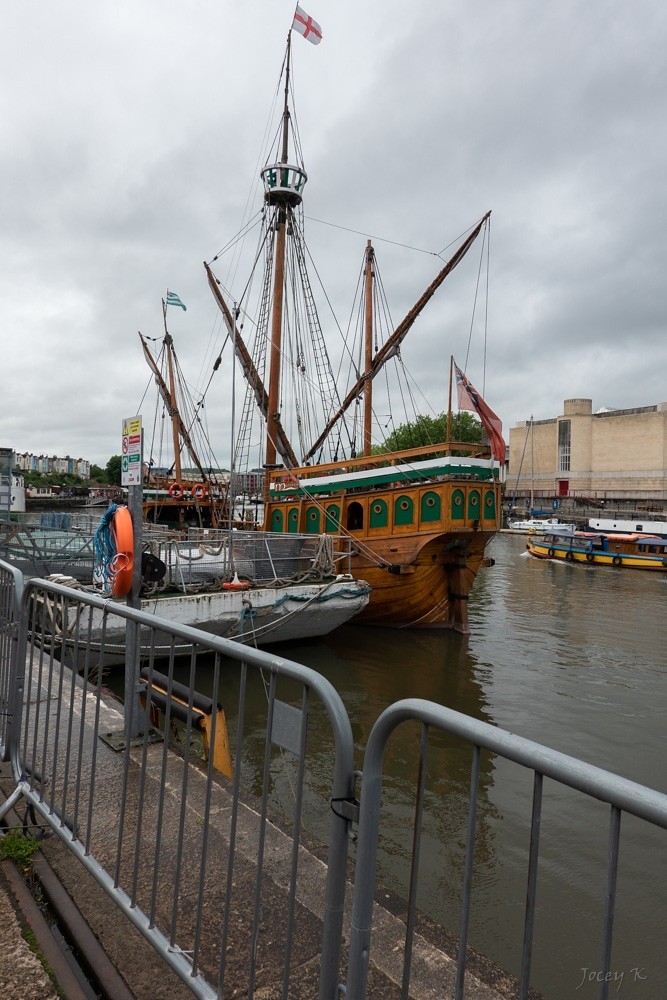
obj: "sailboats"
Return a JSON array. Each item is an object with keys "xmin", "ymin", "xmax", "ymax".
[
  {"xmin": 505, "ymin": 416, "xmax": 577, "ymax": 532},
  {"xmin": 136, "ymin": 1, "xmax": 507, "ymax": 629}
]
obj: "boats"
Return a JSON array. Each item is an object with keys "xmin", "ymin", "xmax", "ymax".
[
  {"xmin": 526, "ymin": 528, "xmax": 667, "ymax": 570},
  {"xmin": 0, "ymin": 512, "xmax": 371, "ymax": 671}
]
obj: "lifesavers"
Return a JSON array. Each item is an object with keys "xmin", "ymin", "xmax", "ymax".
[
  {"xmin": 548, "ymin": 549, "xmax": 555, "ymax": 557},
  {"xmin": 284, "ymin": 477, "xmax": 298, "ymax": 499},
  {"xmin": 586, "ymin": 552, "xmax": 594, "ymax": 561},
  {"xmin": 105, "ymin": 506, "xmax": 134, "ymax": 599},
  {"xmin": 612, "ymin": 556, "xmax": 622, "ymax": 566},
  {"xmin": 192, "ymin": 483, "xmax": 206, "ymax": 500},
  {"xmin": 169, "ymin": 482, "xmax": 184, "ymax": 499},
  {"xmin": 222, "ymin": 582, "xmax": 249, "ymax": 590},
  {"xmin": 566, "ymin": 551, "xmax": 574, "ymax": 562}
]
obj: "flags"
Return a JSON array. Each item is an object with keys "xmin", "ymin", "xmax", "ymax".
[
  {"xmin": 452, "ymin": 359, "xmax": 506, "ymax": 466},
  {"xmin": 166, "ymin": 288, "xmax": 186, "ymax": 312},
  {"xmin": 292, "ymin": 5, "xmax": 322, "ymax": 45}
]
